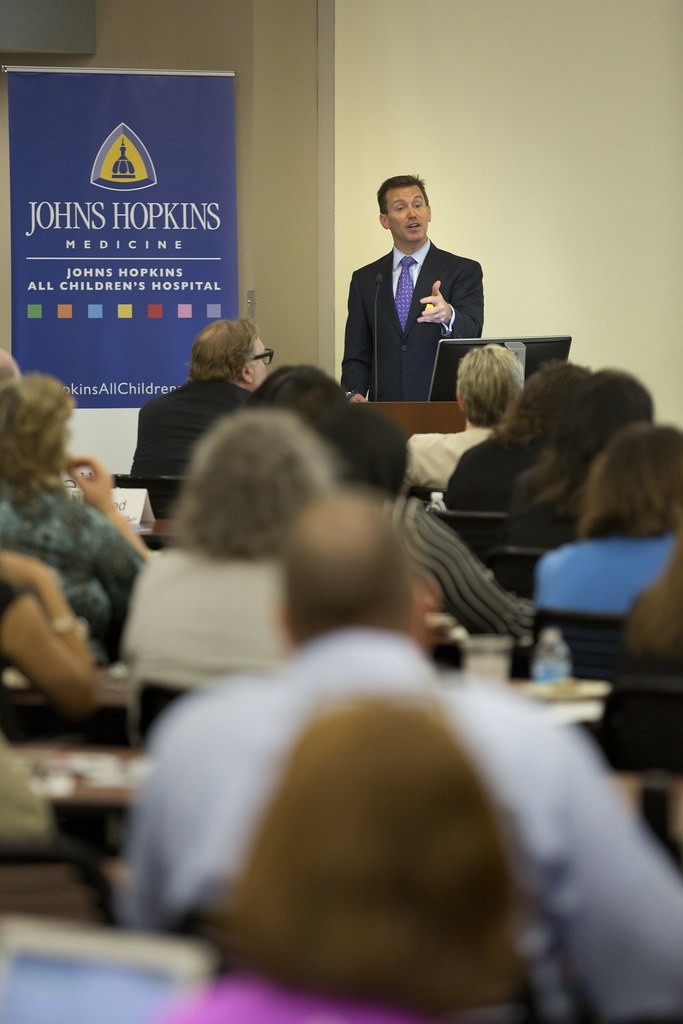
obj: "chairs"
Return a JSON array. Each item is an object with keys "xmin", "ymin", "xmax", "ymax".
[{"xmin": 0, "ymin": 472, "xmax": 683, "ymax": 1024}]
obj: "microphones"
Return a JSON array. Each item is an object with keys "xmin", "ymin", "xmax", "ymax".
[{"xmin": 373, "ymin": 274, "xmax": 382, "ymax": 401}]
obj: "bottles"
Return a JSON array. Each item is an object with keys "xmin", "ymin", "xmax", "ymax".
[
  {"xmin": 427, "ymin": 491, "xmax": 447, "ymax": 513},
  {"xmin": 533, "ymin": 628, "xmax": 571, "ymax": 683}
]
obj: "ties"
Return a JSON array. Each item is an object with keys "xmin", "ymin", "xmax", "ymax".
[{"xmin": 394, "ymin": 256, "xmax": 417, "ymax": 336}]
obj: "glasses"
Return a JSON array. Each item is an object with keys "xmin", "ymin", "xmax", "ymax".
[{"xmin": 249, "ymin": 348, "xmax": 274, "ymax": 365}]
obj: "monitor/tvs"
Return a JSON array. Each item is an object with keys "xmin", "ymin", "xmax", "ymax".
[{"xmin": 428, "ymin": 335, "xmax": 571, "ymax": 403}]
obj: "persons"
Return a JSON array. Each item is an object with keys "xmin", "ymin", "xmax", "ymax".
[
  {"xmin": 338, "ymin": 175, "xmax": 484, "ymax": 397},
  {"xmin": 0, "ymin": 317, "xmax": 683, "ymax": 1024}
]
